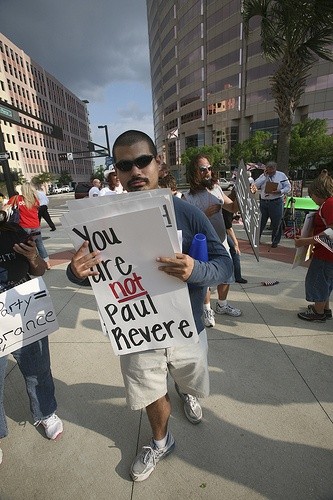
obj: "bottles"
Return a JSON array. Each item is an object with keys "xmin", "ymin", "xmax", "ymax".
[{"xmin": 190, "ymin": 234, "xmax": 209, "ymax": 264}]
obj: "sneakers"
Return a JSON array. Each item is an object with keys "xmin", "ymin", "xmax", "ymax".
[
  {"xmin": 129, "ymin": 430, "xmax": 175, "ymax": 482},
  {"xmin": 203, "ymin": 307, "xmax": 215, "ymax": 328},
  {"xmin": 174, "ymin": 381, "xmax": 203, "ymax": 424},
  {"xmin": 215, "ymin": 301, "xmax": 242, "ymax": 317},
  {"xmin": 297, "ymin": 305, "xmax": 332, "ymax": 322},
  {"xmin": 34, "ymin": 414, "xmax": 64, "ymax": 440}
]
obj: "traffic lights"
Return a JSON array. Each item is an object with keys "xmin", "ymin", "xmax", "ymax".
[{"xmin": 58, "ymin": 153, "xmax": 68, "ymax": 161}]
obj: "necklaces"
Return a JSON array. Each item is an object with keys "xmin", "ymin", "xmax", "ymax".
[{"xmin": 197, "ymin": 189, "xmax": 210, "ymax": 210}]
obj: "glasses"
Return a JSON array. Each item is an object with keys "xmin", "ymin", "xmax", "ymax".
[
  {"xmin": 113, "ymin": 153, "xmax": 156, "ymax": 172},
  {"xmin": 195, "ymin": 165, "xmax": 212, "ymax": 171}
]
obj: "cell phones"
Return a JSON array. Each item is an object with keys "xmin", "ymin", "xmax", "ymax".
[{"xmin": 19, "ymin": 231, "xmax": 41, "ymax": 248}]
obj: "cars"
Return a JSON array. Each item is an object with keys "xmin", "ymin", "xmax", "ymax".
[
  {"xmin": 49, "ymin": 185, "xmax": 75, "ymax": 194},
  {"xmin": 219, "ymin": 178, "xmax": 235, "ymax": 191}
]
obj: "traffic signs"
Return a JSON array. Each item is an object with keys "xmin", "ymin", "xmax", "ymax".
[{"xmin": 0, "ymin": 153, "xmax": 10, "ymax": 159}]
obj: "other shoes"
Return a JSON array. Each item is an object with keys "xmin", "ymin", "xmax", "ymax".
[
  {"xmin": 50, "ymin": 228, "xmax": 57, "ymax": 231},
  {"xmin": 235, "ymin": 277, "xmax": 247, "ymax": 283},
  {"xmin": 272, "ymin": 243, "xmax": 278, "ymax": 248}
]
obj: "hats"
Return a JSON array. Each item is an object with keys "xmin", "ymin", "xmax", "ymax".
[{"xmin": 104, "ymin": 164, "xmax": 115, "ymax": 179}]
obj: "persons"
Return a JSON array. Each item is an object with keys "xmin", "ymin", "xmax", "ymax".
[
  {"xmin": 247, "ymin": 161, "xmax": 292, "ymax": 248},
  {"xmin": 89, "ymin": 179, "xmax": 101, "ymax": 198},
  {"xmin": 66, "ymin": 129, "xmax": 234, "ymax": 482},
  {"xmin": 0, "ymin": 183, "xmax": 56, "ymax": 270},
  {"xmin": 158, "ymin": 174, "xmax": 184, "ymax": 199},
  {"xmin": 99, "ymin": 164, "xmax": 127, "ymax": 197},
  {"xmin": 0, "ymin": 221, "xmax": 64, "ymax": 465},
  {"xmin": 294, "ymin": 174, "xmax": 333, "ymax": 323},
  {"xmin": 180, "ymin": 153, "xmax": 258, "ymax": 328}
]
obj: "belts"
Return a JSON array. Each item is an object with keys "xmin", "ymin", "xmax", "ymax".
[{"xmin": 260, "ymin": 196, "xmax": 281, "ymax": 203}]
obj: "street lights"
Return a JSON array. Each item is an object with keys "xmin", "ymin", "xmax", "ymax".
[{"xmin": 98, "ymin": 125, "xmax": 110, "ymax": 157}]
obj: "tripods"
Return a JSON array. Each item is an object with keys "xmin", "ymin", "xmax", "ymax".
[{"xmin": 274, "ymin": 176, "xmax": 296, "ymax": 239}]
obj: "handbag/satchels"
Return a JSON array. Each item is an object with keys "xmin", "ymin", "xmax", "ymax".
[{"xmin": 9, "ymin": 194, "xmax": 20, "ymax": 224}]
